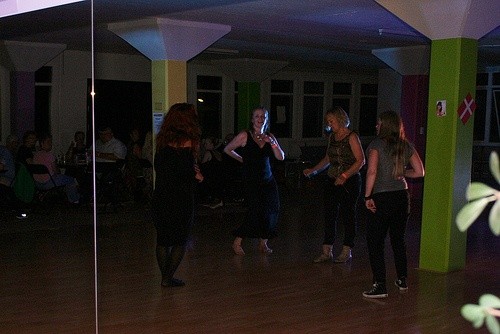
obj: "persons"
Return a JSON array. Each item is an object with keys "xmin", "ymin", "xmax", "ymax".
[
  {"xmin": 0, "ymin": 131, "xmax": 31, "ymax": 223},
  {"xmin": 125, "ymin": 128, "xmax": 153, "ymax": 202},
  {"xmin": 436, "ymin": 100, "xmax": 446, "ymax": 117},
  {"xmin": 150, "ymin": 102, "xmax": 204, "ymax": 288},
  {"xmin": 303, "ymin": 106, "xmax": 366, "ymax": 263},
  {"xmin": 362, "ymin": 110, "xmax": 425, "ymax": 298},
  {"xmin": 18, "ymin": 131, "xmax": 89, "ymax": 203},
  {"xmin": 195, "ymin": 132, "xmax": 246, "ymax": 209},
  {"xmin": 87, "ymin": 126, "xmax": 128, "ymax": 207},
  {"xmin": 224, "ymin": 106, "xmax": 285, "ymax": 256}
]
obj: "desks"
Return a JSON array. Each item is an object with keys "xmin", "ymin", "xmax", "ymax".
[{"xmin": 58, "ymin": 160, "xmax": 87, "ymax": 165}]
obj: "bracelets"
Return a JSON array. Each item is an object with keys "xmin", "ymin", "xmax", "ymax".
[
  {"xmin": 364, "ymin": 195, "xmax": 373, "ymax": 200},
  {"xmin": 271, "ymin": 141, "xmax": 276, "ymax": 146}
]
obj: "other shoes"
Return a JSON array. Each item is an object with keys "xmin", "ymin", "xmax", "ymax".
[
  {"xmin": 204, "ymin": 200, "xmax": 224, "ymax": 209},
  {"xmin": 162, "ymin": 276, "xmax": 185, "ymax": 287}
]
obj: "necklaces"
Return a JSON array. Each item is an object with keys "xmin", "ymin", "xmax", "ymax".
[{"xmin": 254, "ymin": 131, "xmax": 265, "ymax": 142}]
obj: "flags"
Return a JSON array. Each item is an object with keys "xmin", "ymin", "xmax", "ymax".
[{"xmin": 457, "ymin": 93, "xmax": 476, "ymax": 124}]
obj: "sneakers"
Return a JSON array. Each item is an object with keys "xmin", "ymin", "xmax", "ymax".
[
  {"xmin": 363, "ymin": 280, "xmax": 388, "ymax": 298},
  {"xmin": 395, "ymin": 279, "xmax": 408, "ymax": 290}
]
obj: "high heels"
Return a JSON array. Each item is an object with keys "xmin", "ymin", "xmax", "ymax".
[
  {"xmin": 232, "ymin": 238, "xmax": 246, "ymax": 256},
  {"xmin": 335, "ymin": 246, "xmax": 352, "ymax": 262},
  {"xmin": 313, "ymin": 246, "xmax": 334, "ymax": 262},
  {"xmin": 258, "ymin": 239, "xmax": 272, "ymax": 253}
]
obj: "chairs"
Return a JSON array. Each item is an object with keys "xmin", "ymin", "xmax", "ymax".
[
  {"xmin": 26, "ymin": 164, "xmax": 66, "ymax": 211},
  {"xmin": 87, "ymin": 161, "xmax": 119, "ymax": 212}
]
